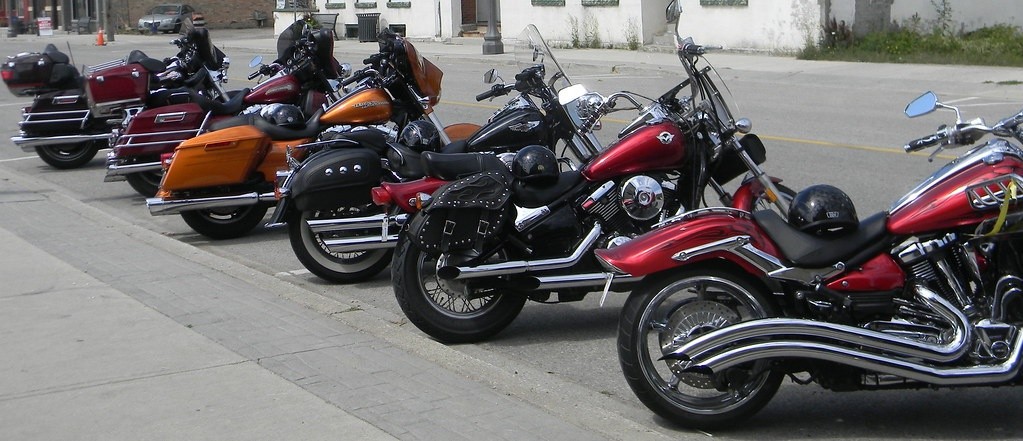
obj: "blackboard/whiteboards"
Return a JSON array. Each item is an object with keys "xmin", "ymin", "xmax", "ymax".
[{"xmin": 37, "ymin": 17, "xmax": 53, "ymax": 35}]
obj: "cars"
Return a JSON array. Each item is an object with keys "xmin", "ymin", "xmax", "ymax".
[{"xmin": 138, "ymin": 3, "xmax": 197, "ymax": 33}]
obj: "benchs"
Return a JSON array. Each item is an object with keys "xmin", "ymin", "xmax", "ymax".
[
  {"xmin": 311, "ymin": 13, "xmax": 339, "ymax": 40},
  {"xmin": 68, "ymin": 17, "xmax": 91, "ymax": 34}
]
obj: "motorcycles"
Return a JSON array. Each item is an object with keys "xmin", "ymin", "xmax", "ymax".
[
  {"xmin": 142, "ymin": 15, "xmax": 483, "ymax": 240},
  {"xmin": 105, "ymin": 12, "xmax": 352, "ymax": 199},
  {"xmin": 263, "ymin": 23, "xmax": 607, "ymax": 285},
  {"xmin": 1, "ymin": 11, "xmax": 249, "ymax": 171},
  {"xmin": 592, "ymin": 90, "xmax": 1022, "ymax": 427},
  {"xmin": 369, "ymin": 0, "xmax": 804, "ymax": 342}
]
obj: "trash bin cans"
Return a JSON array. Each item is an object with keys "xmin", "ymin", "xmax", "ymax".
[{"xmin": 355, "ymin": 13, "xmax": 381, "ymax": 42}]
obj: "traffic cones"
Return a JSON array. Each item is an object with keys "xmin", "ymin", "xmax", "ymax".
[{"xmin": 94, "ymin": 27, "xmax": 108, "ymax": 47}]
